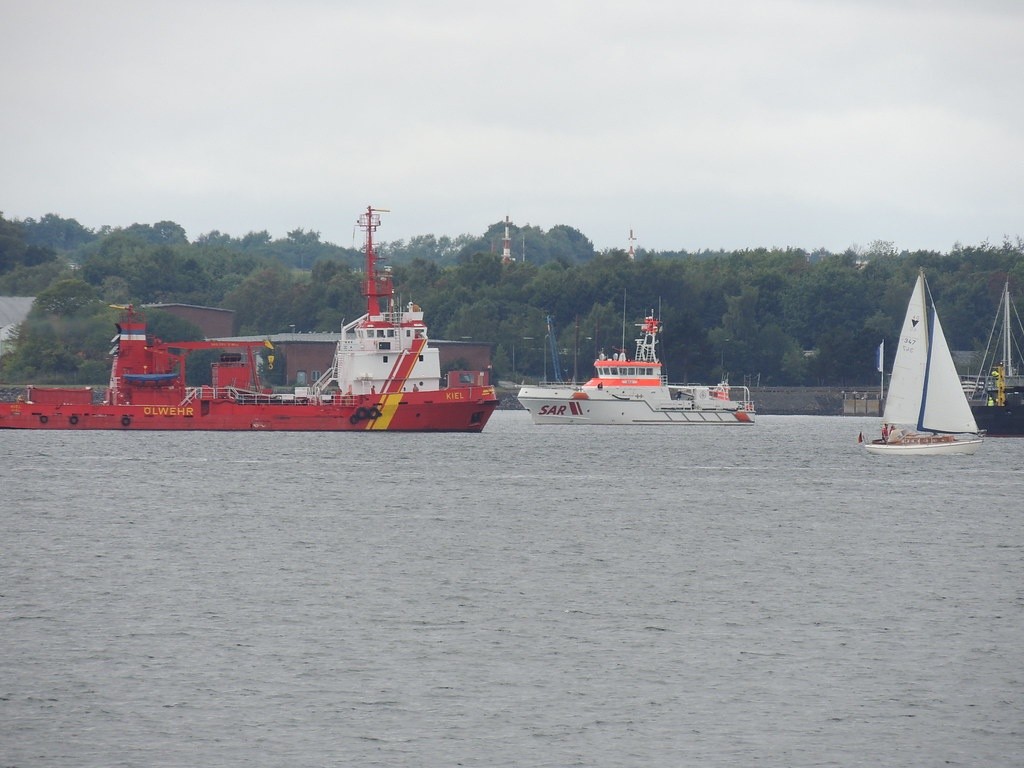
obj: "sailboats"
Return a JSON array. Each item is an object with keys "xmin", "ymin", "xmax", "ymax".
[
  {"xmin": 860, "ymin": 266, "xmax": 988, "ymax": 459},
  {"xmin": 952, "ymin": 273, "xmax": 1024, "ymax": 438}
]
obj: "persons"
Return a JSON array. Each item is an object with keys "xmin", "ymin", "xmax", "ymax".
[
  {"xmin": 597, "ymin": 382, "xmax": 603, "ymax": 389},
  {"xmin": 881, "ymin": 423, "xmax": 889, "ymax": 446},
  {"xmin": 988, "ymin": 397, "xmax": 994, "ymax": 406},
  {"xmin": 413, "ymin": 384, "xmax": 419, "ymax": 392},
  {"xmin": 370, "ymin": 385, "xmax": 375, "ymax": 394}
]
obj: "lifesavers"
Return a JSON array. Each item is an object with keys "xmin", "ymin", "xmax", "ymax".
[
  {"xmin": 349, "ymin": 415, "xmax": 358, "ymax": 424},
  {"xmin": 40, "ymin": 415, "xmax": 48, "ymax": 423},
  {"xmin": 120, "ymin": 417, "xmax": 130, "ymax": 425},
  {"xmin": 369, "ymin": 406, "xmax": 379, "ymax": 419},
  {"xmin": 356, "ymin": 407, "xmax": 369, "ymax": 419},
  {"xmin": 69, "ymin": 416, "xmax": 78, "ymax": 424}
]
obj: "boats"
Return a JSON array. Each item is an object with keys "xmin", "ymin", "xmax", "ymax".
[
  {"xmin": 123, "ymin": 373, "xmax": 178, "ymax": 388},
  {"xmin": 0, "ymin": 206, "xmax": 498, "ymax": 433},
  {"xmin": 515, "ymin": 289, "xmax": 757, "ymax": 427}
]
